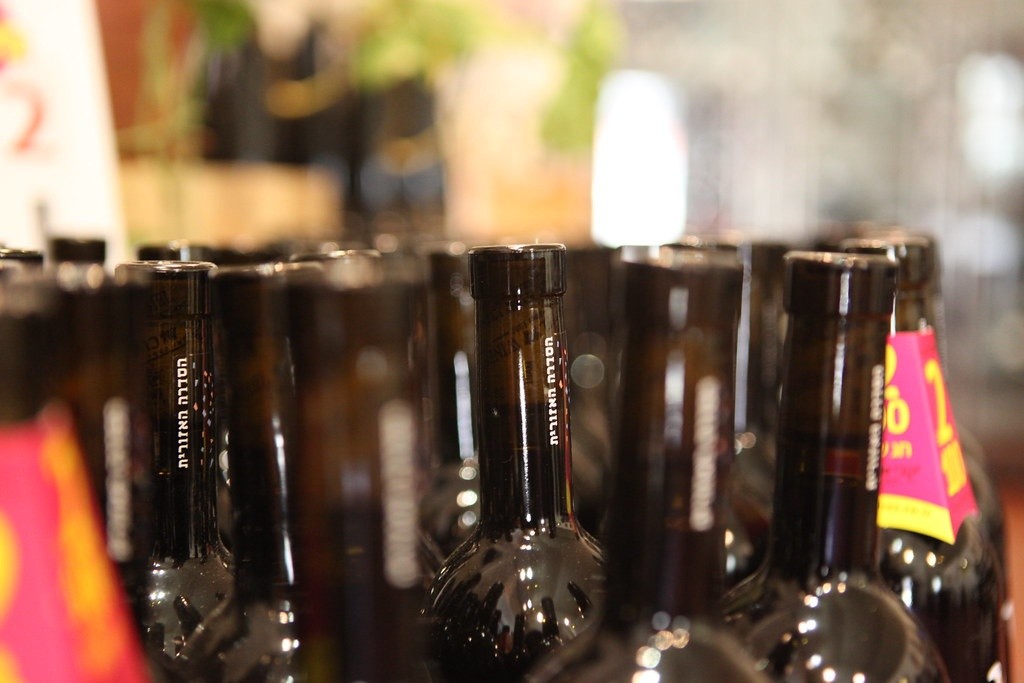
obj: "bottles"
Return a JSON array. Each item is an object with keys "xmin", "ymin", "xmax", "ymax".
[
  {"xmin": 0, "ymin": 234, "xmax": 1016, "ymax": 683},
  {"xmin": 197, "ymin": 2, "xmax": 446, "ymax": 241}
]
